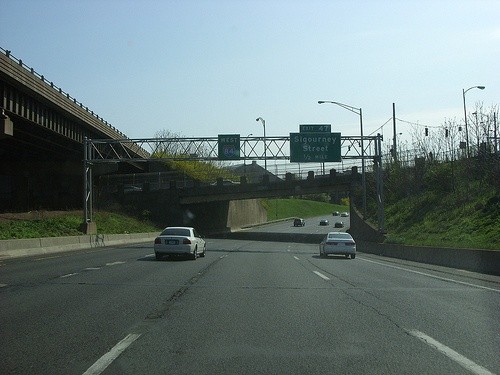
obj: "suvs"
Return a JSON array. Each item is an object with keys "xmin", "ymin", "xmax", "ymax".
[{"xmin": 293, "ymin": 218, "xmax": 305, "ymax": 227}]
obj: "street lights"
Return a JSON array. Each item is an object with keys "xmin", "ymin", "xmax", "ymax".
[
  {"xmin": 462, "ymin": 85, "xmax": 486, "ymax": 158},
  {"xmin": 255, "ymin": 116, "xmax": 267, "ymax": 169},
  {"xmin": 318, "ymin": 100, "xmax": 367, "ymax": 221},
  {"xmin": 244, "ymin": 134, "xmax": 253, "ymax": 165}
]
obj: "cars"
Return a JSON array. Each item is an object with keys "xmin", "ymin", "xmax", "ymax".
[
  {"xmin": 320, "ymin": 218, "xmax": 329, "ymax": 226},
  {"xmin": 154, "ymin": 226, "xmax": 207, "ymax": 260},
  {"xmin": 341, "ymin": 212, "xmax": 349, "ymax": 217},
  {"xmin": 334, "ymin": 221, "xmax": 345, "ymax": 228},
  {"xmin": 319, "ymin": 231, "xmax": 356, "ymax": 259},
  {"xmin": 332, "ymin": 211, "xmax": 340, "ymax": 216}
]
